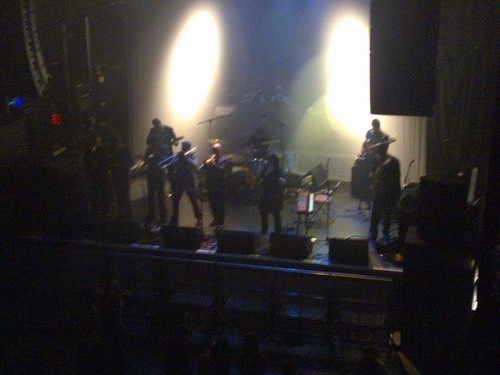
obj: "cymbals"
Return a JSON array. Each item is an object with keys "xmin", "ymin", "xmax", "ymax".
[{"xmin": 262, "ymin": 139, "xmax": 281, "ymax": 146}]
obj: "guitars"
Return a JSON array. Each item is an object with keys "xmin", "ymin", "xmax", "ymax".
[
  {"xmin": 362, "ymin": 138, "xmax": 396, "ymax": 157},
  {"xmin": 145, "ymin": 137, "xmax": 184, "ymax": 155}
]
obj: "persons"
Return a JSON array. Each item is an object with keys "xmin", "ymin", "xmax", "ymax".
[
  {"xmin": 87, "ymin": 135, "xmax": 131, "ymax": 223},
  {"xmin": 146, "ymin": 118, "xmax": 177, "ymax": 182},
  {"xmin": 146, "ymin": 134, "xmax": 169, "ymax": 224},
  {"xmin": 248, "ymin": 127, "xmax": 271, "ymax": 159},
  {"xmin": 363, "ymin": 119, "xmax": 389, "ymax": 152},
  {"xmin": 256, "ymin": 155, "xmax": 283, "ymax": 233},
  {"xmin": 370, "ymin": 139, "xmax": 401, "ymax": 242},
  {"xmin": 171, "ymin": 141, "xmax": 203, "ymax": 227},
  {"xmin": 205, "ymin": 144, "xmax": 225, "ymax": 226}
]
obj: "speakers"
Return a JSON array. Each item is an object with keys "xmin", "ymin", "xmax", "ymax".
[
  {"xmin": 367, "ymin": 0, "xmax": 441, "ymax": 115},
  {"xmin": 269, "ymin": 231, "xmax": 312, "ymax": 261},
  {"xmin": 328, "ymin": 238, "xmax": 369, "ymax": 266},
  {"xmin": 217, "ymin": 228, "xmax": 261, "ymax": 255},
  {"xmin": 100, "ymin": 220, "xmax": 144, "ymax": 245},
  {"xmin": 160, "ymin": 223, "xmax": 207, "ymax": 250}
]
